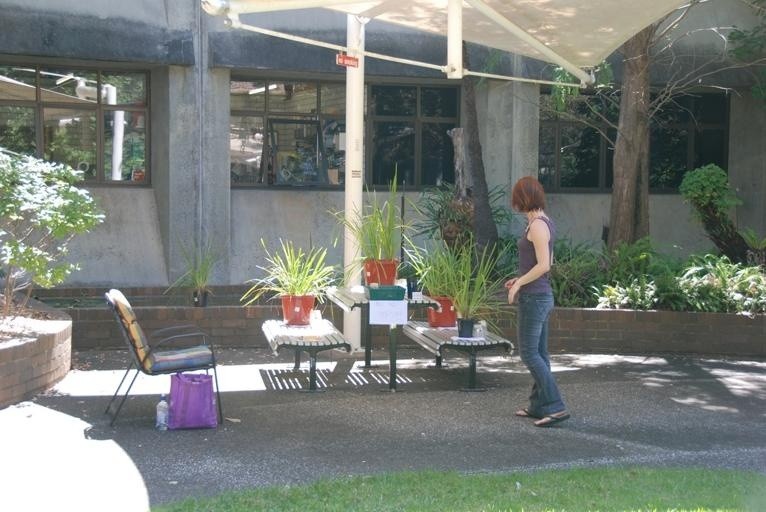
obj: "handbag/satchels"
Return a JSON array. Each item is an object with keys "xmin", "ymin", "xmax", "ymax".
[{"xmin": 169, "ymin": 374, "xmax": 219, "ymax": 432}]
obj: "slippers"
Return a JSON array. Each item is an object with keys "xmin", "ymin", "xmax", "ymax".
[{"xmin": 518, "ymin": 405, "xmax": 571, "ymax": 427}]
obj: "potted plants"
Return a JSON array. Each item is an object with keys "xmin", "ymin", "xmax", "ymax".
[
  {"xmin": 161, "ymin": 230, "xmax": 234, "ymax": 307},
  {"xmin": 401, "ymin": 226, "xmax": 478, "ymax": 327},
  {"xmin": 239, "ymin": 234, "xmax": 344, "ymax": 326},
  {"xmin": 427, "ymin": 233, "xmax": 520, "ymax": 337},
  {"xmin": 324, "ymin": 162, "xmax": 423, "ymax": 285}
]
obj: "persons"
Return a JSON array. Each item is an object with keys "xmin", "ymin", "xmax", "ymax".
[{"xmin": 505, "ymin": 176, "xmax": 571, "ymax": 427}]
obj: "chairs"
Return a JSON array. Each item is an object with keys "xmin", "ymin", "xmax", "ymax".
[{"xmin": 104, "ymin": 288, "xmax": 226, "ymax": 428}]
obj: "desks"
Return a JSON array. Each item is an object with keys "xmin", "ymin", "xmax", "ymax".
[{"xmin": 326, "ymin": 284, "xmax": 441, "ymax": 393}]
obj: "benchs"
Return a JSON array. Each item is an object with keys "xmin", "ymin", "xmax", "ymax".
[
  {"xmin": 261, "ymin": 318, "xmax": 352, "ymax": 394},
  {"xmin": 403, "ymin": 320, "xmax": 515, "ymax": 392}
]
